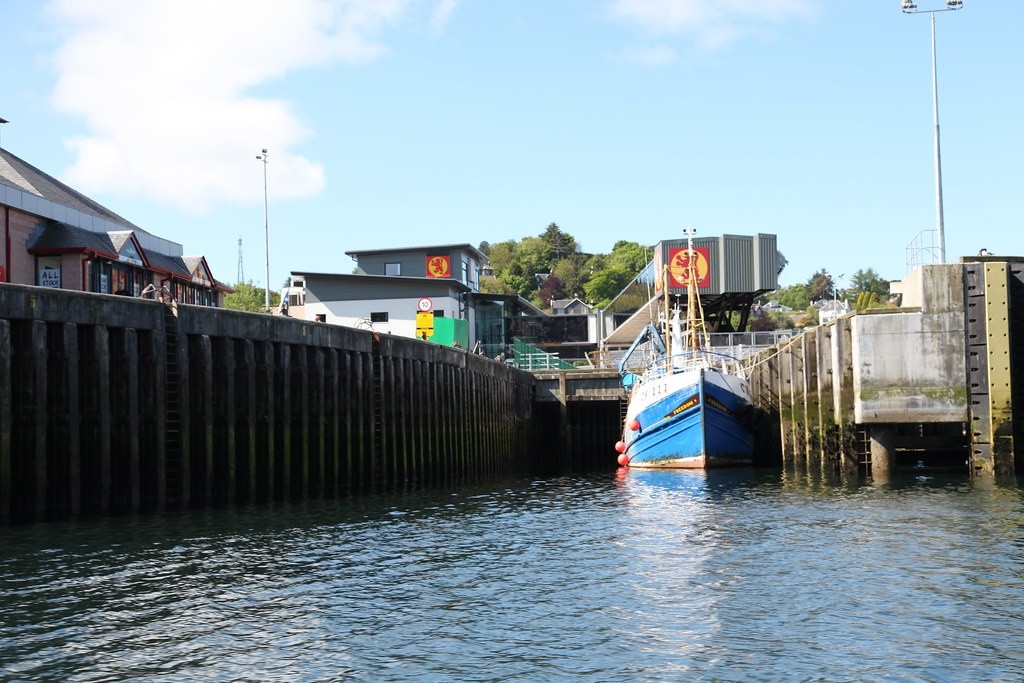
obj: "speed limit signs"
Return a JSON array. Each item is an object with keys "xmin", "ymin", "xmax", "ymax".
[{"xmin": 418, "ymin": 297, "xmax": 432, "ymax": 312}]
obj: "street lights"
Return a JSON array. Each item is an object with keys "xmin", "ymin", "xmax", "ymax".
[
  {"xmin": 901, "ymin": 0, "xmax": 963, "ymax": 263},
  {"xmin": 256, "ymin": 149, "xmax": 270, "ymax": 311}
]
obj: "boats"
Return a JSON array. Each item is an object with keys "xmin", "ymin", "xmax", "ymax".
[{"xmin": 618, "ymin": 224, "xmax": 765, "ymax": 470}]
